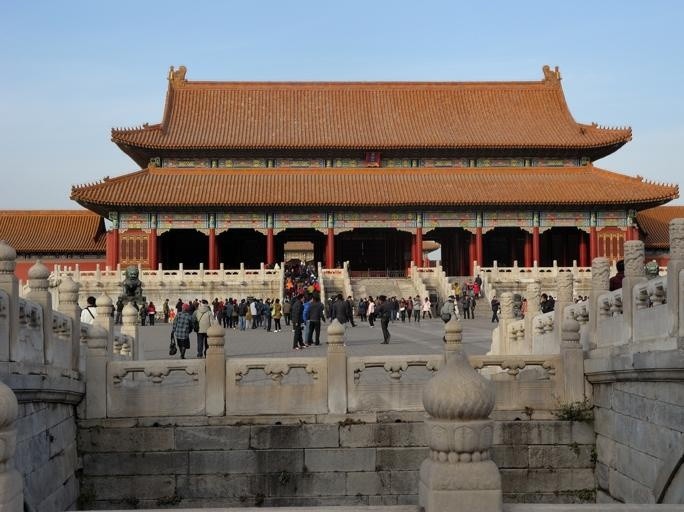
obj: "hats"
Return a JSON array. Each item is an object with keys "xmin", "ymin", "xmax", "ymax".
[{"xmin": 182, "ymin": 304, "xmax": 189, "ymax": 311}]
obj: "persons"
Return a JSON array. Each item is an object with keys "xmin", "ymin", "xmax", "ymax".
[
  {"xmin": 163, "ymin": 299, "xmax": 202, "ymax": 323},
  {"xmin": 133, "ymin": 301, "xmax": 155, "ymax": 327},
  {"xmin": 212, "ymin": 297, "xmax": 291, "ymax": 332},
  {"xmin": 113, "ymin": 298, "xmax": 124, "ymax": 324},
  {"xmin": 191, "ymin": 299, "xmax": 215, "ymax": 358},
  {"xmin": 265, "ymin": 261, "xmax": 276, "ymax": 274},
  {"xmin": 490, "ymin": 295, "xmax": 500, "ymax": 323},
  {"xmin": 325, "ymin": 296, "xmax": 337, "ymax": 319},
  {"xmin": 307, "ymin": 295, "xmax": 326, "ymax": 345},
  {"xmin": 518, "ymin": 293, "xmax": 590, "ymax": 321},
  {"xmin": 331, "ymin": 293, "xmax": 350, "ymax": 347},
  {"xmin": 302, "ymin": 296, "xmax": 313, "ymax": 347},
  {"xmin": 374, "ymin": 295, "xmax": 393, "ymax": 345},
  {"xmin": 452, "ymin": 274, "xmax": 483, "ymax": 319},
  {"xmin": 345, "ymin": 295, "xmax": 381, "ymax": 327},
  {"xmin": 388, "ymin": 295, "xmax": 432, "ymax": 322},
  {"xmin": 170, "ymin": 303, "xmax": 196, "ymax": 360},
  {"xmin": 439, "ymin": 294, "xmax": 460, "ymax": 343},
  {"xmin": 78, "ymin": 295, "xmax": 100, "ymax": 326},
  {"xmin": 283, "ymin": 262, "xmax": 320, "ymax": 303},
  {"xmin": 609, "ymin": 259, "xmax": 624, "ymax": 291},
  {"xmin": 289, "ymin": 293, "xmax": 310, "ymax": 351}
]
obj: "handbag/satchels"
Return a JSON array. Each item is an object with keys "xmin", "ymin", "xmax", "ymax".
[
  {"xmin": 170, "ymin": 343, "xmax": 177, "ymax": 355},
  {"xmin": 194, "ymin": 321, "xmax": 199, "ymax": 332}
]
